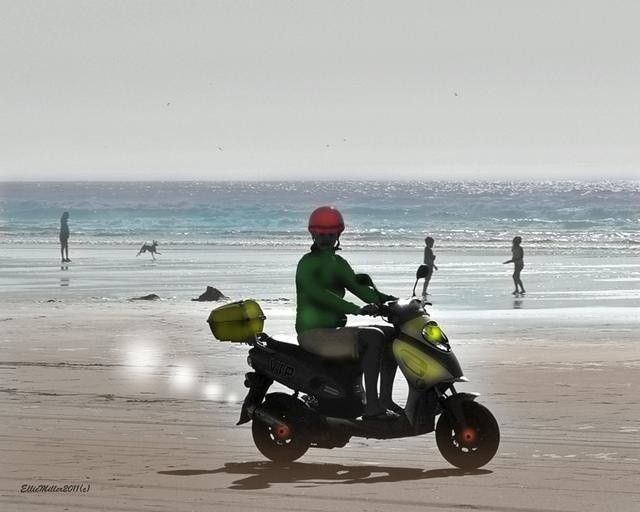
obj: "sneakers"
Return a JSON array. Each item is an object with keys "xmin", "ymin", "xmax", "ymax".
[{"xmin": 363, "ymin": 400, "xmax": 407, "ymax": 421}]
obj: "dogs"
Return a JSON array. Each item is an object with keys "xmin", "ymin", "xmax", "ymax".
[{"xmin": 136, "ymin": 240, "xmax": 162, "ymax": 261}]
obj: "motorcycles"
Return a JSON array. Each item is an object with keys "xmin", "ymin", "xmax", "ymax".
[{"xmin": 205, "ymin": 263, "xmax": 503, "ymax": 473}]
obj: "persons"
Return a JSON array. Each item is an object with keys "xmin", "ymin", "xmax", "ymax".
[
  {"xmin": 58, "ymin": 210, "xmax": 72, "ymax": 262},
  {"xmin": 421, "ymin": 236, "xmax": 438, "ymax": 297},
  {"xmin": 294, "ymin": 205, "xmax": 405, "ymax": 427},
  {"xmin": 502, "ymin": 236, "xmax": 527, "ymax": 293}
]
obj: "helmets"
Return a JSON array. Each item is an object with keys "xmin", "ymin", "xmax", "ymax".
[{"xmin": 307, "ymin": 206, "xmax": 344, "ymax": 235}]
